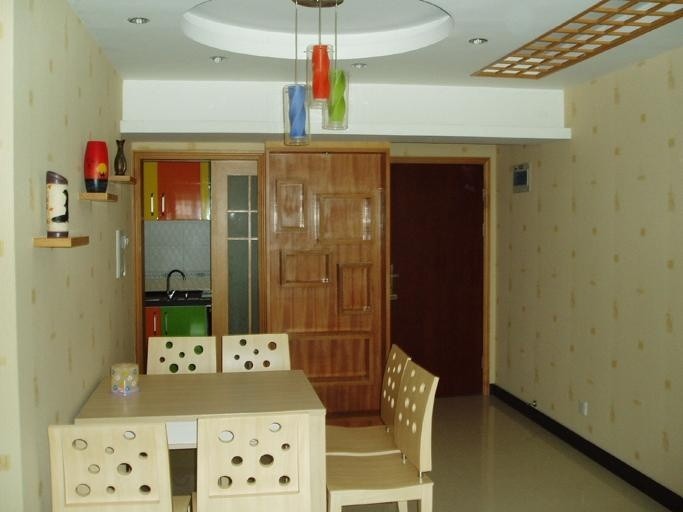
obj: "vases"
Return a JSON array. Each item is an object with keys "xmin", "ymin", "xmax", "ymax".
[
  {"xmin": 114, "ymin": 139, "xmax": 127, "ymax": 175},
  {"xmin": 83, "ymin": 142, "xmax": 108, "ymax": 192}
]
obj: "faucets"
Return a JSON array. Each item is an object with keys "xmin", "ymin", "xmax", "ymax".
[{"xmin": 167, "ymin": 270, "xmax": 185, "ymax": 299}]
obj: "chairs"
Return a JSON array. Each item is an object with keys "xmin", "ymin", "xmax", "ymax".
[
  {"xmin": 326, "ymin": 344, "xmax": 411, "ymax": 457},
  {"xmin": 146, "ymin": 337, "xmax": 216, "ymax": 375},
  {"xmin": 326, "ymin": 360, "xmax": 439, "ymax": 511},
  {"xmin": 47, "ymin": 420, "xmax": 190, "ymax": 512},
  {"xmin": 192, "ymin": 410, "xmax": 326, "ymax": 511},
  {"xmin": 221, "ymin": 334, "xmax": 291, "ymax": 372}
]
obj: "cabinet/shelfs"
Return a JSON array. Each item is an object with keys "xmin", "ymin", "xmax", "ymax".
[
  {"xmin": 144, "ymin": 305, "xmax": 207, "ymax": 372},
  {"xmin": 142, "ymin": 160, "xmax": 210, "ymax": 221}
]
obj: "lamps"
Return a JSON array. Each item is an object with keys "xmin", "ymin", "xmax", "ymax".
[{"xmin": 283, "ymin": 1, "xmax": 349, "ymax": 147}]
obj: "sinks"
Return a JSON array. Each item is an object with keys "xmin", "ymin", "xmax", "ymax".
[{"xmin": 170, "ymin": 289, "xmax": 201, "ymax": 303}]
